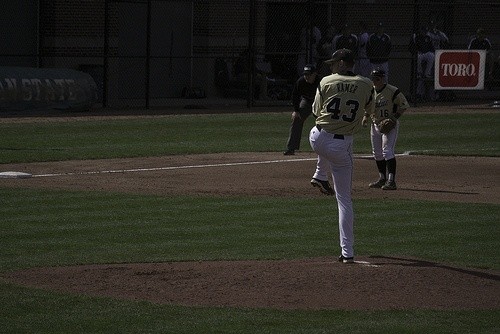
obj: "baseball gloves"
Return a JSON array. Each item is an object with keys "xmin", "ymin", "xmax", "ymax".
[{"xmin": 378, "ymin": 119, "xmax": 396, "ymax": 135}]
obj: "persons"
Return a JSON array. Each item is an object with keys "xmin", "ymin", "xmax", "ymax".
[
  {"xmin": 363, "ymin": 67, "xmax": 410, "ymax": 190},
  {"xmin": 284, "ymin": 67, "xmax": 321, "ymax": 155},
  {"xmin": 309, "ymin": 48, "xmax": 375, "ymax": 264},
  {"xmin": 236, "ymin": 18, "xmax": 500, "ymax": 107}
]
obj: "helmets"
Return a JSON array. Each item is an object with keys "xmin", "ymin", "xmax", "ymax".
[{"xmin": 371, "ymin": 66, "xmax": 386, "ymax": 76}]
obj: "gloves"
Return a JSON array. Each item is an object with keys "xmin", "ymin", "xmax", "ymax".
[{"xmin": 362, "ymin": 116, "xmax": 368, "ymax": 128}]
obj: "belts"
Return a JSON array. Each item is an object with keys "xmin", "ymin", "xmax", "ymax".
[{"xmin": 316, "ymin": 124, "xmax": 345, "ymax": 140}]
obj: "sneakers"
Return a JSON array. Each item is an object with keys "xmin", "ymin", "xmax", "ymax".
[
  {"xmin": 311, "ymin": 177, "xmax": 336, "ymax": 196},
  {"xmin": 283, "ymin": 150, "xmax": 294, "ymax": 156},
  {"xmin": 337, "ymin": 254, "xmax": 355, "ymax": 263}
]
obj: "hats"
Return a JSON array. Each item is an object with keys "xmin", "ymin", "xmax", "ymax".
[
  {"xmin": 374, "ymin": 21, "xmax": 384, "ymax": 29},
  {"xmin": 343, "ymin": 23, "xmax": 351, "ymax": 29},
  {"xmin": 299, "ymin": 65, "xmax": 317, "ymax": 76},
  {"xmin": 324, "ymin": 47, "xmax": 355, "ymax": 64}
]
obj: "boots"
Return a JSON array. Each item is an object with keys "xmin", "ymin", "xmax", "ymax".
[{"xmin": 368, "ymin": 158, "xmax": 399, "ymax": 191}]
